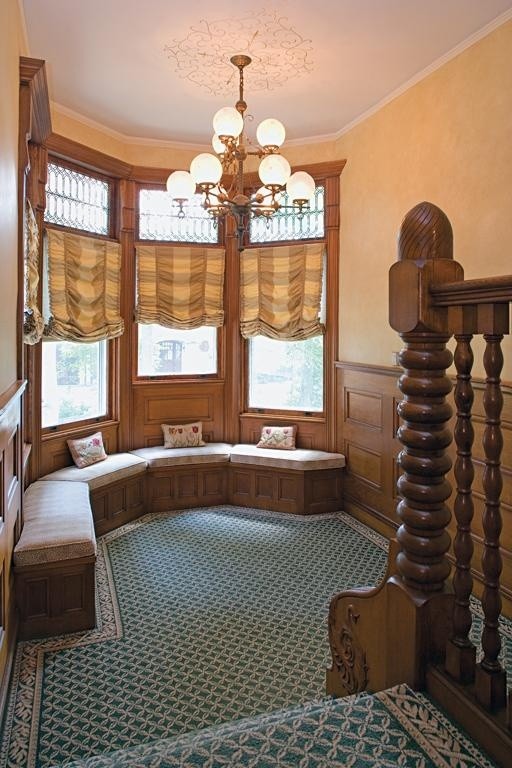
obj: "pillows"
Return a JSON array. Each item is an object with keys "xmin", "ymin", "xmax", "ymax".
[
  {"xmin": 67, "ymin": 431, "xmax": 111, "ymax": 467},
  {"xmin": 255, "ymin": 423, "xmax": 300, "ymax": 448},
  {"xmin": 162, "ymin": 422, "xmax": 205, "ymax": 448}
]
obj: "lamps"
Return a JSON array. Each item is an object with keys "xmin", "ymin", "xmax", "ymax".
[{"xmin": 166, "ymin": 54, "xmax": 330, "ymax": 255}]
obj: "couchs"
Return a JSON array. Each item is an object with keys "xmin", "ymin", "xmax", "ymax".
[
  {"xmin": 131, "ymin": 440, "xmax": 345, "ymax": 518},
  {"xmin": 9, "ymin": 443, "xmax": 145, "ymax": 641}
]
